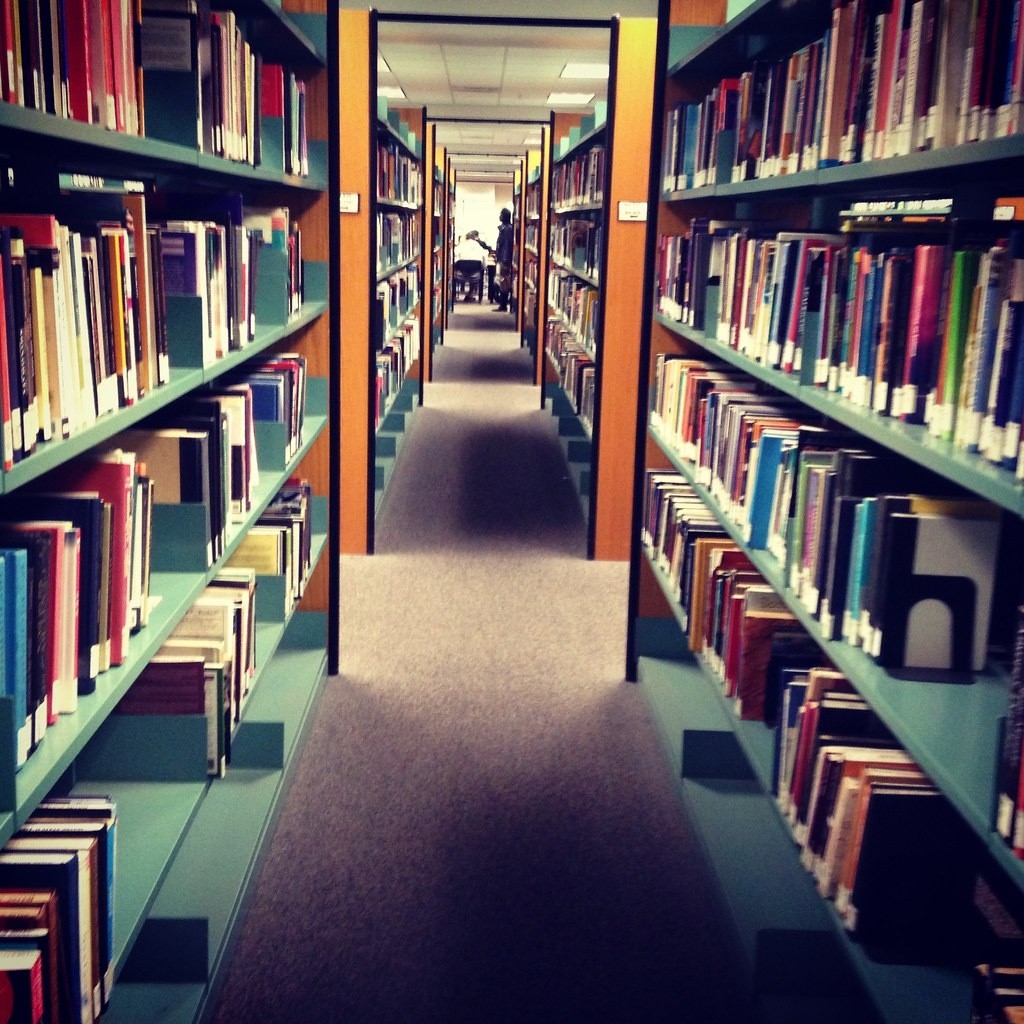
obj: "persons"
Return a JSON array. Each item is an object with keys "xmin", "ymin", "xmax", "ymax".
[{"xmin": 452, "ymin": 209, "xmax": 514, "ymax": 312}]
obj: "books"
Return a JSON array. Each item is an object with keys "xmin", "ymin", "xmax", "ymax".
[
  {"xmin": 1, "ymin": 0, "xmax": 455, "ymax": 1024},
  {"xmin": 515, "ymin": 1, "xmax": 1024, "ymax": 1024}
]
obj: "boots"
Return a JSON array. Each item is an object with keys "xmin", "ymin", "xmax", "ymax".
[{"xmin": 491, "ymin": 291, "xmax": 508, "ymax": 311}]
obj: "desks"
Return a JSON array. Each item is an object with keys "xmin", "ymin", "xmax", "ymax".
[{"xmin": 487, "ymin": 265, "xmax": 500, "ymax": 304}]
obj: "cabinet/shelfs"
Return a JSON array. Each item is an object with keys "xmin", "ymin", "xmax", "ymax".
[{"xmin": 0, "ymin": 0, "xmax": 1024, "ymax": 1024}]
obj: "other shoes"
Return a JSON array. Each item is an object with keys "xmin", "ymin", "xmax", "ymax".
[{"xmin": 464, "ymin": 296, "xmax": 476, "ymax": 301}]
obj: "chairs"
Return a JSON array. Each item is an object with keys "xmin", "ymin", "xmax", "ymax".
[{"xmin": 455, "ymin": 259, "xmax": 484, "ymax": 305}]
